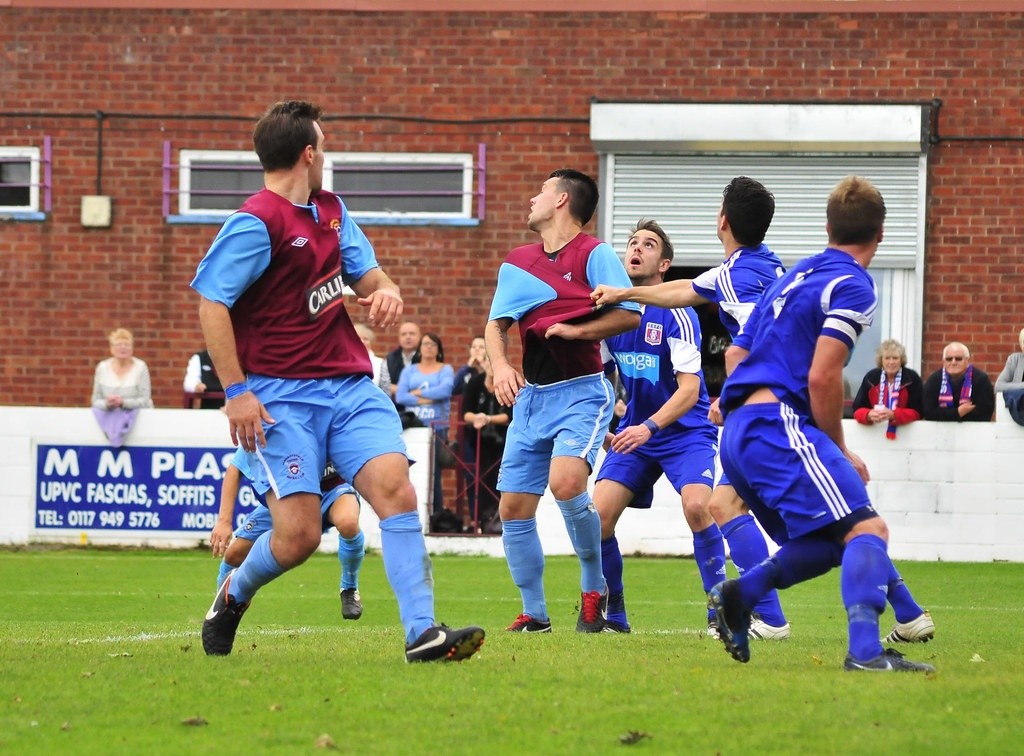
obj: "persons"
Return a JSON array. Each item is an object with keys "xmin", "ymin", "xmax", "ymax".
[
  {"xmin": 452, "ymin": 335, "xmax": 514, "ymax": 535},
  {"xmin": 209, "ymin": 441, "xmax": 367, "ymax": 622},
  {"xmin": 381, "ymin": 320, "xmax": 455, "ymax": 534},
  {"xmin": 482, "ymin": 167, "xmax": 643, "ymax": 633},
  {"xmin": 89, "ymin": 327, "xmax": 155, "ymax": 410},
  {"xmin": 354, "ymin": 324, "xmax": 391, "ymax": 398},
  {"xmin": 706, "ymin": 174, "xmax": 936, "ymax": 676},
  {"xmin": 589, "ymin": 174, "xmax": 935, "ymax": 645},
  {"xmin": 181, "ymin": 349, "xmax": 226, "ymax": 409},
  {"xmin": 189, "ymin": 98, "xmax": 485, "ymax": 664},
  {"xmin": 590, "ymin": 216, "xmax": 735, "ymax": 641},
  {"xmin": 853, "ymin": 326, "xmax": 1024, "ymax": 440}
]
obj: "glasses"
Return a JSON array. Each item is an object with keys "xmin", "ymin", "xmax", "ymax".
[{"xmin": 944, "ymin": 355, "xmax": 966, "ymax": 361}]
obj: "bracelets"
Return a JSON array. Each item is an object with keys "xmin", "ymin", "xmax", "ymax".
[{"xmin": 641, "ymin": 419, "xmax": 660, "ymax": 437}]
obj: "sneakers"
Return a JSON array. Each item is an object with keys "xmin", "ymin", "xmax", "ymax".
[
  {"xmin": 576, "ymin": 580, "xmax": 609, "ymax": 633},
  {"xmin": 603, "ymin": 619, "xmax": 631, "ymax": 633},
  {"xmin": 340, "ymin": 586, "xmax": 362, "ymax": 619},
  {"xmin": 708, "ymin": 579, "xmax": 755, "ymax": 663},
  {"xmin": 845, "ymin": 648, "xmax": 935, "ymax": 675},
  {"xmin": 201, "ymin": 567, "xmax": 252, "ymax": 656},
  {"xmin": 748, "ymin": 619, "xmax": 791, "ymax": 640},
  {"xmin": 707, "ymin": 618, "xmax": 721, "ymax": 636},
  {"xmin": 405, "ymin": 623, "xmax": 486, "ymax": 662},
  {"xmin": 505, "ymin": 612, "xmax": 551, "ymax": 634},
  {"xmin": 880, "ymin": 609, "xmax": 935, "ymax": 643}
]
